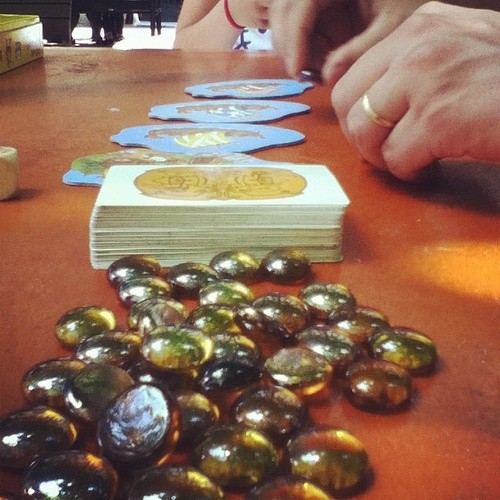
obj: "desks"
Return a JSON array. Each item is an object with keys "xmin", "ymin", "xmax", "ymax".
[{"xmin": 0, "ymin": 48, "xmax": 498, "ymax": 499}]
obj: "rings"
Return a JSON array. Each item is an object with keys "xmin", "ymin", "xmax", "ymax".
[{"xmin": 361, "ymin": 93, "xmax": 395, "ymax": 129}]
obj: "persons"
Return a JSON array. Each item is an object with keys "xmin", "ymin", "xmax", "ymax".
[
  {"xmin": 85, "ymin": 0, "xmax": 126, "ymax": 44},
  {"xmin": 173, "ymin": 0, "xmax": 499, "ymax": 182},
  {"xmin": 150, "ymin": 0, "xmax": 162, "ymax": 36}
]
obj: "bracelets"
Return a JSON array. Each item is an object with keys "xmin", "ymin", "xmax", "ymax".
[{"xmin": 223, "ymin": 0, "xmax": 246, "ymax": 30}]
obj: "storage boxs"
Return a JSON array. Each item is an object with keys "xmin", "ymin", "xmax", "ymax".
[{"xmin": 0, "ymin": 14, "xmax": 44, "ymax": 75}]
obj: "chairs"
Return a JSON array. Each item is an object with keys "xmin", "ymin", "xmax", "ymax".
[{"xmin": 108, "ymin": 0, "xmax": 162, "ymax": 36}]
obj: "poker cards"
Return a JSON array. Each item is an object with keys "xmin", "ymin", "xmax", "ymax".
[{"xmin": 88, "ymin": 162, "xmax": 352, "ymax": 271}]
